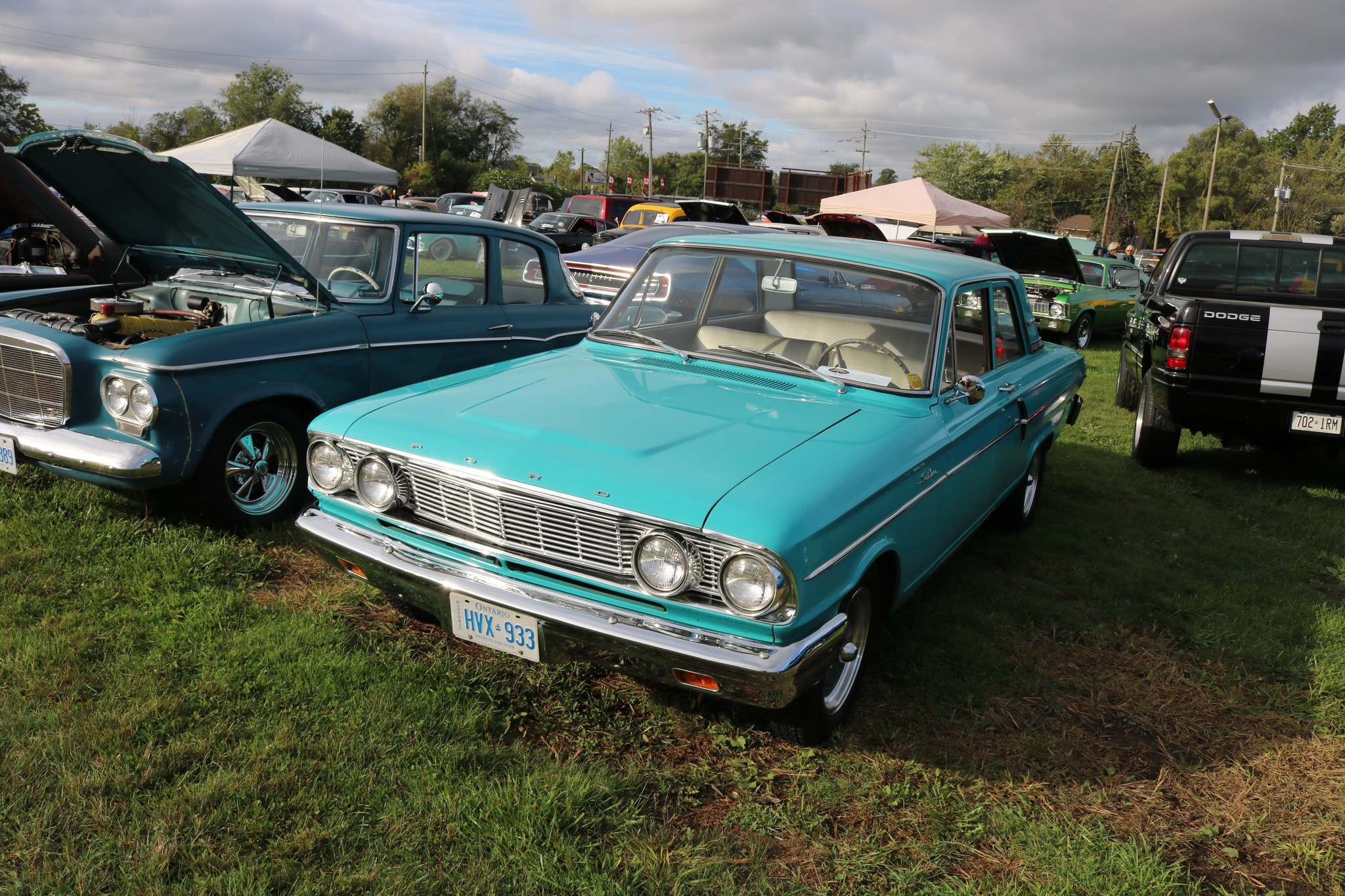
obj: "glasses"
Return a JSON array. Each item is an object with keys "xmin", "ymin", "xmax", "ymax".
[
  {"xmin": 409, "ymin": 193, "xmax": 411, "ymax": 194},
  {"xmin": 1128, "ymin": 250, "xmax": 1132, "ymax": 252}
]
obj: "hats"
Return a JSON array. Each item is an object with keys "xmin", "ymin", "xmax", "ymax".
[{"xmin": 1098, "ymin": 250, "xmax": 1103, "ymax": 252}]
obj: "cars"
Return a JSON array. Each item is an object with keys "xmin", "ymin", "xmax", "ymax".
[
  {"xmin": 501, "ymin": 197, "xmax": 1169, "ymax": 349},
  {"xmin": 0, "ymin": 129, "xmax": 666, "ymax": 542},
  {"xmin": 293, "ymin": 234, "xmax": 1088, "ymax": 748},
  {"xmin": 211, "ymin": 184, "xmax": 568, "ymax": 261}
]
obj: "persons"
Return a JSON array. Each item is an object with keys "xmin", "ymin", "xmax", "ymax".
[
  {"xmin": 1102, "ymin": 242, "xmax": 1120, "ymax": 259},
  {"xmin": 1119, "ymin": 245, "xmax": 1135, "ymax": 265},
  {"xmin": 390, "ymin": 189, "xmax": 400, "ymax": 200},
  {"xmin": 1098, "ymin": 250, "xmax": 1103, "ymax": 257},
  {"xmin": 377, "ymin": 186, "xmax": 391, "ymax": 200},
  {"xmin": 403, "ymin": 189, "xmax": 415, "ymax": 199}
]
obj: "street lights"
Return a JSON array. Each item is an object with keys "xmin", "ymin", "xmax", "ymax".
[{"xmin": 1198, "ymin": 99, "xmax": 1232, "ymax": 258}]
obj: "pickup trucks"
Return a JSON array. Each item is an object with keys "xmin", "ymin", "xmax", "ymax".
[{"xmin": 1114, "ymin": 230, "xmax": 1345, "ymax": 468}]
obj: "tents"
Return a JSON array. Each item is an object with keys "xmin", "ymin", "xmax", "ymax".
[
  {"xmin": 817, "ymin": 177, "xmax": 1010, "ymax": 245},
  {"xmin": 155, "ymin": 117, "xmax": 399, "ymax": 207}
]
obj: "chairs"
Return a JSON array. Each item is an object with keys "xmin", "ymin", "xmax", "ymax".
[
  {"xmin": 395, "ymin": 285, "xmax": 481, "ymax": 308},
  {"xmin": 830, "ymin": 340, "xmax": 950, "ymax": 395},
  {"xmin": 556, "ymin": 220, "xmax": 567, "ymax": 231},
  {"xmin": 686, "ymin": 323, "xmax": 828, "ymax": 371},
  {"xmin": 1204, "ymin": 256, "xmax": 1345, "ymax": 300}
]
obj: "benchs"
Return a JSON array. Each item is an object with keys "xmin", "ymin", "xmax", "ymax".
[
  {"xmin": 752, "ymin": 302, "xmax": 991, "ymax": 371},
  {"xmin": 368, "ymin": 259, "xmax": 544, "ymax": 314}
]
